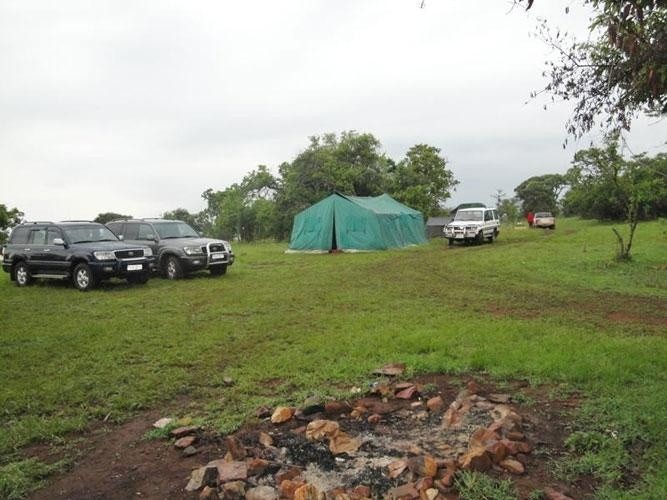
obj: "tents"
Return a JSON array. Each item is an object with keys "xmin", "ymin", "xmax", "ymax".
[{"xmin": 285, "ymin": 189, "xmax": 430, "ymax": 253}]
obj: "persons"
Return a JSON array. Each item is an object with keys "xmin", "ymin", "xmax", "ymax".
[{"xmin": 528, "ymin": 209, "xmax": 534, "ymax": 228}]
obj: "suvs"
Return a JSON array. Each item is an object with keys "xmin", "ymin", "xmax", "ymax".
[
  {"xmin": 104, "ymin": 219, "xmax": 234, "ymax": 280},
  {"xmin": 2, "ymin": 218, "xmax": 158, "ymax": 292},
  {"xmin": 443, "ymin": 208, "xmax": 500, "ymax": 246}
]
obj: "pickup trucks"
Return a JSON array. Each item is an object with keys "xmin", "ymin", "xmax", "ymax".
[{"xmin": 534, "ymin": 212, "xmax": 554, "ymax": 227}]
